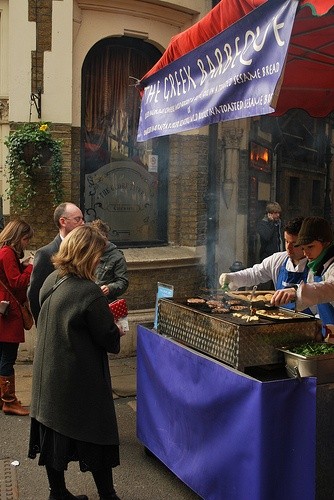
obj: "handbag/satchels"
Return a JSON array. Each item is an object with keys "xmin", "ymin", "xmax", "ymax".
[{"xmin": 19, "ymin": 301, "xmax": 33, "ymax": 330}]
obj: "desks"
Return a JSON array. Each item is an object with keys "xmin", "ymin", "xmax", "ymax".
[{"xmin": 136, "ymin": 323, "xmax": 318, "ymax": 500}]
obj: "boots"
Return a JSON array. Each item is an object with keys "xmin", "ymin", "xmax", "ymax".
[{"xmin": 0, "ymin": 374, "xmax": 30, "ymax": 415}]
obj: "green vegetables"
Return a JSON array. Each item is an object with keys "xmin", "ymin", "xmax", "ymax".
[{"xmin": 285, "ymin": 340, "xmax": 334, "ymax": 357}]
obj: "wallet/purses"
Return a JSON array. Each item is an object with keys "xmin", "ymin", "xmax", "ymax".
[{"xmin": 108, "ymin": 299, "xmax": 129, "ymax": 321}]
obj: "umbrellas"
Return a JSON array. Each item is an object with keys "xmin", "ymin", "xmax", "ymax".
[{"xmin": 134, "ymin": 0, "xmax": 334, "ymax": 142}]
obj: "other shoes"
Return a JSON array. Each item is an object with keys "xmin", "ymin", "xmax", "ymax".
[{"xmin": 49, "ymin": 490, "xmax": 89, "ymax": 500}]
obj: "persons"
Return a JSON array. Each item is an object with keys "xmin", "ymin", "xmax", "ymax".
[
  {"xmin": 0, "ymin": 219, "xmax": 36, "ymax": 416},
  {"xmin": 87, "ymin": 220, "xmax": 130, "ymax": 302},
  {"xmin": 26, "ymin": 202, "xmax": 82, "ymax": 328},
  {"xmin": 28, "ymin": 225, "xmax": 124, "ymax": 500},
  {"xmin": 258, "ymin": 201, "xmax": 286, "ymax": 291},
  {"xmin": 272, "ymin": 213, "xmax": 334, "ymax": 343},
  {"xmin": 220, "ymin": 217, "xmax": 317, "ymax": 317}
]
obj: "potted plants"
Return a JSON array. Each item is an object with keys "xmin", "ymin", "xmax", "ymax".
[{"xmin": 3, "ymin": 122, "xmax": 66, "ymax": 217}]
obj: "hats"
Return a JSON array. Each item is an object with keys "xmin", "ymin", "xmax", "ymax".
[{"xmin": 293, "ymin": 216, "xmax": 334, "ymax": 248}]
